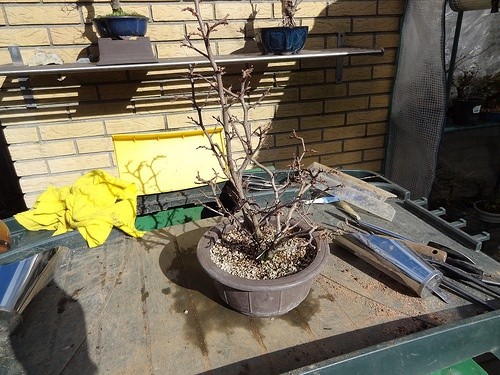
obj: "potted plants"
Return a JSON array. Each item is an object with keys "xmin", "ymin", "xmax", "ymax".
[
  {"xmin": 426, "ymin": 61, "xmax": 499, "ymax": 225},
  {"xmin": 168, "ymin": 0, "xmax": 345, "ymax": 318},
  {"xmin": 254, "ymin": 0, "xmax": 309, "ymax": 56},
  {"xmin": 91, "ymin": 0, "xmax": 151, "ymax": 39}
]
